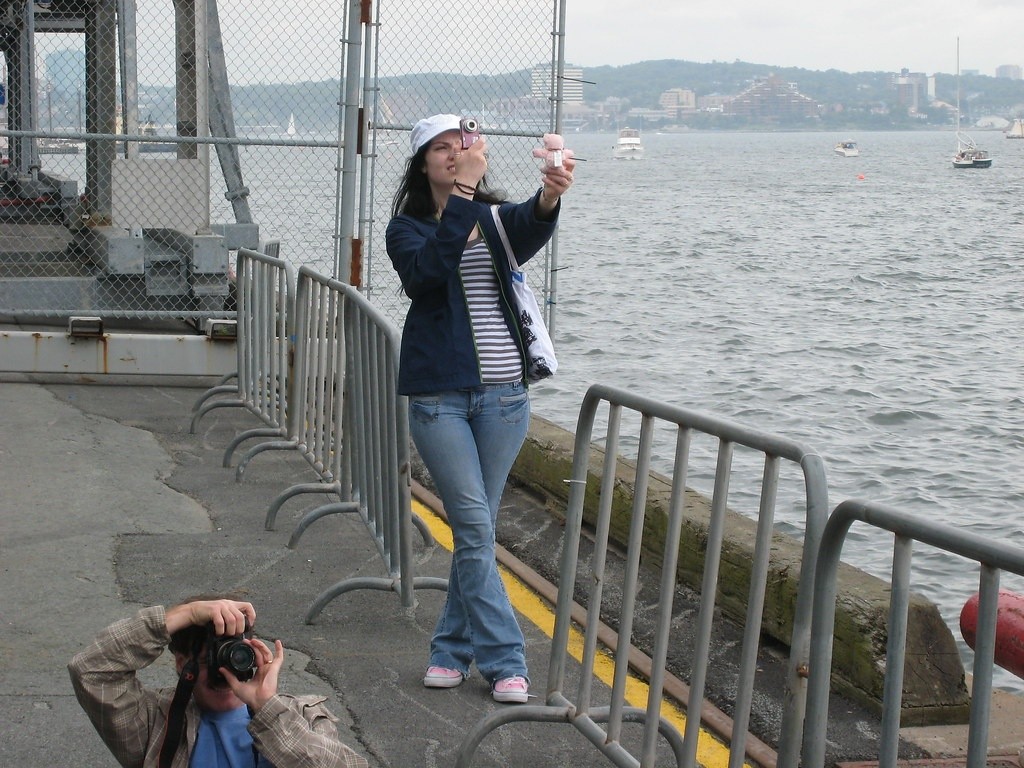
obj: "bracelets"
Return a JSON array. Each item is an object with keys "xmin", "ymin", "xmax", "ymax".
[
  {"xmin": 543, "ymin": 190, "xmax": 558, "ymax": 202},
  {"xmin": 451, "ymin": 179, "xmax": 478, "ymax": 195}
]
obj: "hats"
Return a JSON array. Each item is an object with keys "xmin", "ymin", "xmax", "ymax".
[
  {"xmin": 409, "ymin": 113, "xmax": 462, "ymax": 156},
  {"xmin": 168, "ymin": 595, "xmax": 254, "ymax": 653}
]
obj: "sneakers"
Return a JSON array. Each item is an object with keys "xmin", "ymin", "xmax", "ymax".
[
  {"xmin": 492, "ymin": 676, "xmax": 528, "ymax": 703},
  {"xmin": 424, "ymin": 666, "xmax": 464, "ymax": 687}
]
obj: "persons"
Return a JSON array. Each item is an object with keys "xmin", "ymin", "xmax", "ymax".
[
  {"xmin": 386, "ymin": 112, "xmax": 575, "ymax": 704},
  {"xmin": 66, "ymin": 590, "xmax": 369, "ymax": 768}
]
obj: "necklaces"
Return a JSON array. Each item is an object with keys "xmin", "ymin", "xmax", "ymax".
[{"xmin": 437, "ymin": 209, "xmax": 442, "ymax": 218}]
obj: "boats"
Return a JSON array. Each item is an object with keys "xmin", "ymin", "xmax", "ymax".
[
  {"xmin": 1005, "ymin": 118, "xmax": 1023, "ymax": 140},
  {"xmin": 612, "ymin": 118, "xmax": 644, "ymax": 161},
  {"xmin": 834, "ymin": 139, "xmax": 860, "ymax": 157}
]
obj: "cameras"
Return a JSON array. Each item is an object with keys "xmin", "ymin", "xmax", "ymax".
[
  {"xmin": 206, "ymin": 614, "xmax": 257, "ymax": 689},
  {"xmin": 460, "ymin": 119, "xmax": 479, "ymax": 149}
]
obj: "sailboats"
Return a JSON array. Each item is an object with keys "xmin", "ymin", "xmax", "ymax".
[{"xmin": 952, "ymin": 35, "xmax": 994, "ymax": 169}]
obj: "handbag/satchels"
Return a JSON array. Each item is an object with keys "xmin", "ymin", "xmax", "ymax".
[{"xmin": 491, "ymin": 204, "xmax": 558, "ymax": 383}]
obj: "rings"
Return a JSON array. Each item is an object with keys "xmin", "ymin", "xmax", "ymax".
[
  {"xmin": 265, "ymin": 661, "xmax": 273, "ymax": 664},
  {"xmin": 569, "ymin": 176, "xmax": 573, "ymax": 181},
  {"xmin": 564, "ymin": 182, "xmax": 568, "ymax": 186}
]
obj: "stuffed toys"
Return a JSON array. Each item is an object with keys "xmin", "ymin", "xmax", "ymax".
[{"xmin": 532, "ymin": 132, "xmax": 573, "ymax": 174}]
